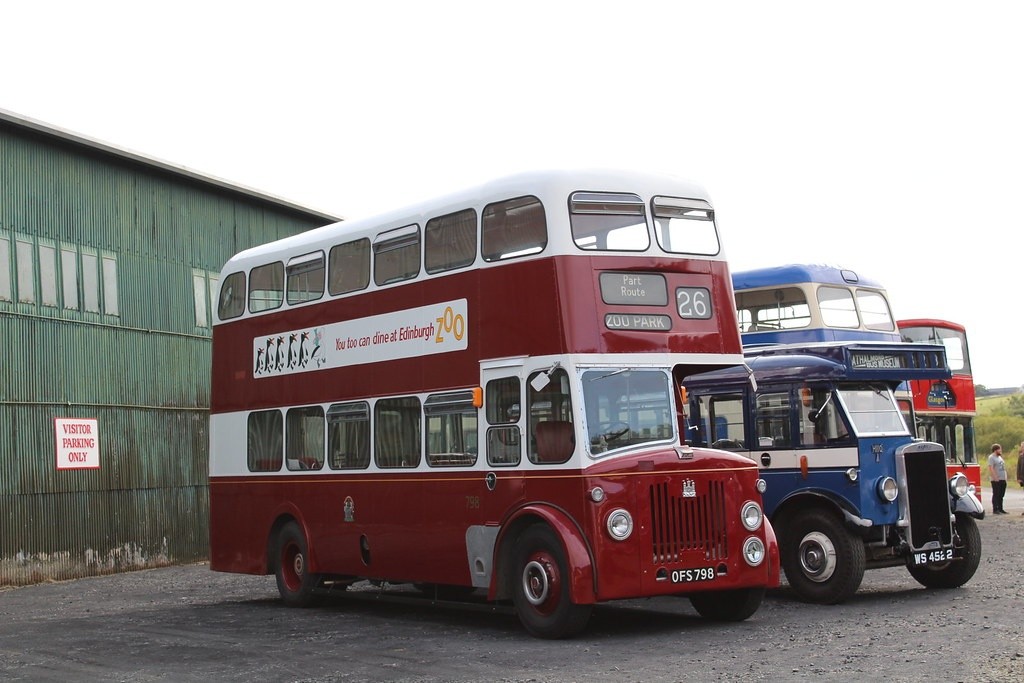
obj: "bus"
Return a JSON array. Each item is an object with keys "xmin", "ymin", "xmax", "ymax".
[
  {"xmin": 209, "ymin": 175, "xmax": 781, "ymax": 641},
  {"xmin": 890, "ymin": 319, "xmax": 983, "ymax": 502},
  {"xmin": 532, "ymin": 262, "xmax": 986, "ymax": 605}
]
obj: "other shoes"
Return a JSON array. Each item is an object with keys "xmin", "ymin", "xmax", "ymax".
[
  {"xmin": 993, "ymin": 510, "xmax": 1001, "ymax": 515},
  {"xmin": 1021, "ymin": 512, "xmax": 1024, "ymax": 515},
  {"xmin": 1000, "ymin": 510, "xmax": 1008, "ymax": 514}
]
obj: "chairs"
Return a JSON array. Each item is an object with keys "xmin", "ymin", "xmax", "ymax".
[
  {"xmin": 535, "ymin": 419, "xmax": 579, "ymax": 462},
  {"xmin": 295, "ymin": 456, "xmax": 318, "ymax": 471},
  {"xmin": 797, "ymin": 431, "xmax": 829, "ymax": 446},
  {"xmin": 248, "ymin": 458, "xmax": 284, "ymax": 469}
]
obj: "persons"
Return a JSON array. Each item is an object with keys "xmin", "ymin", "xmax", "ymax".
[
  {"xmin": 988, "ymin": 444, "xmax": 1009, "ymax": 514},
  {"xmin": 1017, "ymin": 442, "xmax": 1024, "ymax": 488}
]
